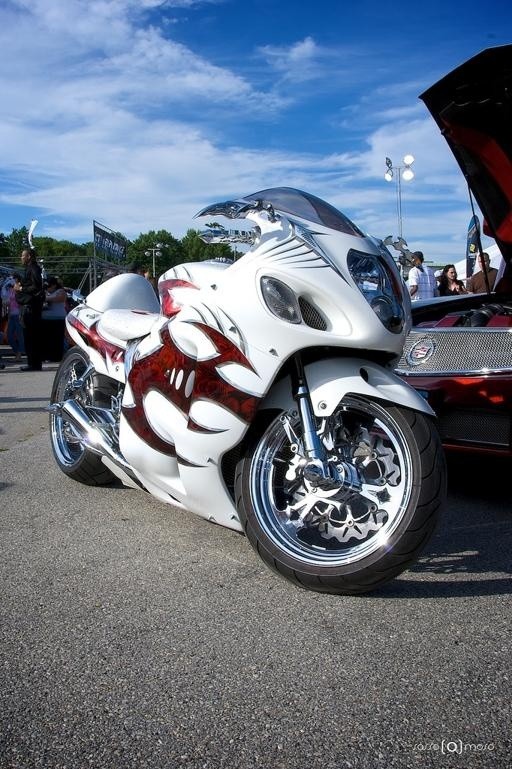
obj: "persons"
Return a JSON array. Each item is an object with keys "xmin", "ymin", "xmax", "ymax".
[
  {"xmin": 409, "ymin": 251, "xmax": 498, "ymax": 302},
  {"xmin": 0, "ymin": 271, "xmax": 68, "ymax": 371},
  {"xmin": 14, "ymin": 248, "xmax": 46, "ymax": 371},
  {"xmin": 127, "ymin": 260, "xmax": 158, "ymax": 297}
]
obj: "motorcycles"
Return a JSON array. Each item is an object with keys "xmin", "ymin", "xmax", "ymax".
[{"xmin": 42, "ymin": 183, "xmax": 443, "ymax": 599}]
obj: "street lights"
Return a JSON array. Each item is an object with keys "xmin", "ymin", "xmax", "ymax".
[
  {"xmin": 382, "ymin": 152, "xmax": 416, "ymax": 278},
  {"xmin": 143, "ymin": 245, "xmax": 163, "ymax": 278}
]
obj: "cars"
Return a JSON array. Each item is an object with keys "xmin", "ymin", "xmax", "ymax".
[{"xmin": 384, "ymin": 40, "xmax": 512, "ymax": 497}]
